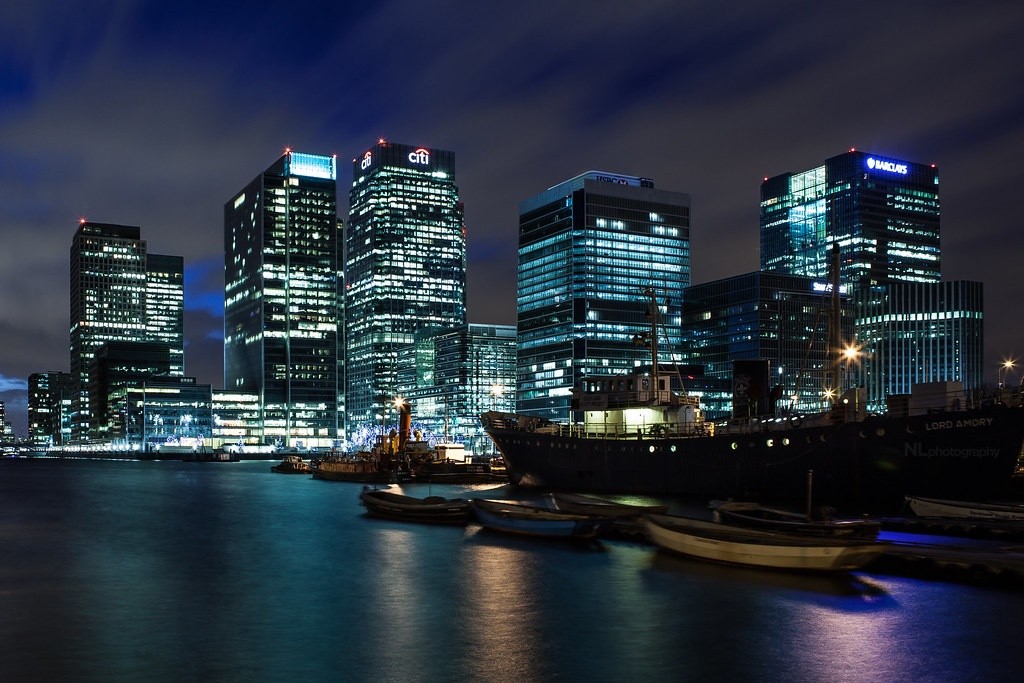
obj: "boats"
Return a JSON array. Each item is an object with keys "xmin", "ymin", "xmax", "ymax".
[
  {"xmin": 270, "ymin": 239, "xmax": 1024, "ymax": 580},
  {"xmin": 25, "ymin": 451, "xmax": 47, "ymax": 458}
]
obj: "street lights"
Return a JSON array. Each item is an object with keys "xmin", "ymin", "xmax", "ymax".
[
  {"xmin": 997, "ymin": 360, "xmax": 1013, "ymax": 403},
  {"xmin": 845, "ymin": 343, "xmax": 860, "ymax": 390}
]
